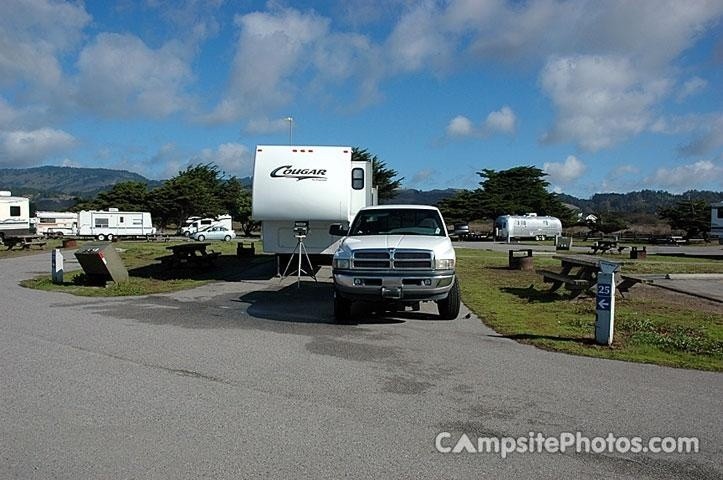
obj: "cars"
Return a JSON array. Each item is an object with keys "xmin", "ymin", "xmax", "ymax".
[
  {"xmin": 189, "ymin": 225, "xmax": 236, "ymax": 241},
  {"xmin": 454, "ymin": 225, "xmax": 469, "ymax": 236},
  {"xmin": 329, "ymin": 204, "xmax": 461, "ymax": 320}
]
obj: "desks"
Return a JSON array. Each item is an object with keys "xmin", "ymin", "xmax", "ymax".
[
  {"xmin": 594, "ymin": 240, "xmax": 620, "ymax": 255},
  {"xmin": 551, "ymin": 254, "xmax": 639, "ymax": 301},
  {"xmin": 167, "ymin": 243, "xmax": 211, "ymax": 269}
]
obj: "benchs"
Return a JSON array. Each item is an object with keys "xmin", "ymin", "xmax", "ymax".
[
  {"xmin": 591, "ymin": 246, "xmax": 629, "ymax": 255},
  {"xmin": 536, "ymin": 268, "xmax": 654, "ymax": 303},
  {"xmin": 155, "ymin": 252, "xmax": 220, "ymax": 268}
]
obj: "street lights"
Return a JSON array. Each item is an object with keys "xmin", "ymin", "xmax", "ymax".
[{"xmin": 280, "ymin": 113, "xmax": 293, "ymax": 145}]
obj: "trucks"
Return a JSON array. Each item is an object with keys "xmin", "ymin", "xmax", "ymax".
[{"xmin": 181, "ymin": 214, "xmax": 232, "ymax": 236}]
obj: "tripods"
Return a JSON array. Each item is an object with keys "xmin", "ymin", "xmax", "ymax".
[{"xmin": 280, "ymin": 236, "xmax": 319, "ymax": 288}]
